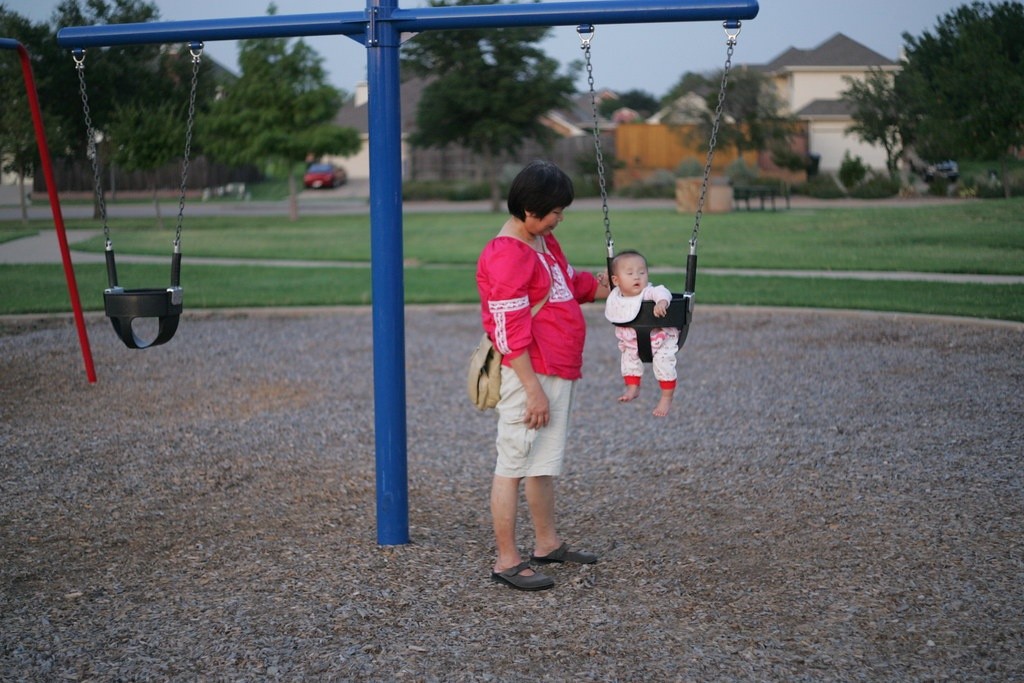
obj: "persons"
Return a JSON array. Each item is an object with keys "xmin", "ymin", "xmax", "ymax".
[
  {"xmin": 475, "ymin": 161, "xmax": 610, "ymax": 590},
  {"xmin": 597, "ymin": 250, "xmax": 680, "ymax": 417}
]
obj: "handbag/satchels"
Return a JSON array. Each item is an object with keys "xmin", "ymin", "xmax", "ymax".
[{"xmin": 466, "ymin": 332, "xmax": 503, "ymax": 411}]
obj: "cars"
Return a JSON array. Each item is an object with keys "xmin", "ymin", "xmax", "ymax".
[
  {"xmin": 926, "ymin": 160, "xmax": 958, "ymax": 183},
  {"xmin": 302, "ymin": 163, "xmax": 348, "ymax": 191}
]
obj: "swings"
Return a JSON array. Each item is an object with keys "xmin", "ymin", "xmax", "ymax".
[
  {"xmin": 576, "ymin": 19, "xmax": 743, "ymax": 364},
  {"xmin": 68, "ymin": 39, "xmax": 206, "ymax": 352}
]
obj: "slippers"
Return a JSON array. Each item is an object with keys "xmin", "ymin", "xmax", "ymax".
[
  {"xmin": 489, "ymin": 562, "xmax": 555, "ymax": 590},
  {"xmin": 529, "ymin": 541, "xmax": 598, "ymax": 566}
]
